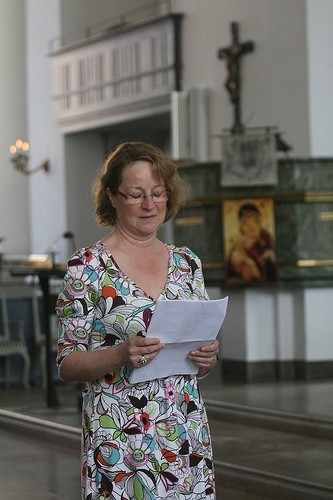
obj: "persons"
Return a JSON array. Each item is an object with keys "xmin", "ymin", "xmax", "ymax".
[{"xmin": 55, "ymin": 139, "xmax": 219, "ymax": 500}]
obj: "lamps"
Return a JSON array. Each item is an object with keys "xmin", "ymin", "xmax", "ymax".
[{"xmin": 9, "ymin": 139, "xmax": 49, "ymax": 175}]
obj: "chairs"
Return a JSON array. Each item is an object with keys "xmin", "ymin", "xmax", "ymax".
[
  {"xmin": 0, "ymin": 291, "xmax": 32, "ymax": 392},
  {"xmin": 34, "ymin": 268, "xmax": 83, "ymax": 410}
]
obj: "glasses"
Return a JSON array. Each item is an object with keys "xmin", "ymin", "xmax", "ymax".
[{"xmin": 109, "ymin": 186, "xmax": 172, "ymax": 203}]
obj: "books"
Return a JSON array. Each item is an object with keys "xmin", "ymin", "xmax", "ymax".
[{"xmin": 1, "ymin": 254, "xmax": 67, "ymax": 271}]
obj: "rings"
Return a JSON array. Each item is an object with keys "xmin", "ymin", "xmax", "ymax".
[{"xmin": 140, "ymin": 355, "xmax": 147, "ymax": 365}]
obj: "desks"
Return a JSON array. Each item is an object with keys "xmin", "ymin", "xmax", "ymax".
[{"xmin": 0, "ymin": 283, "xmax": 48, "ymax": 392}]
